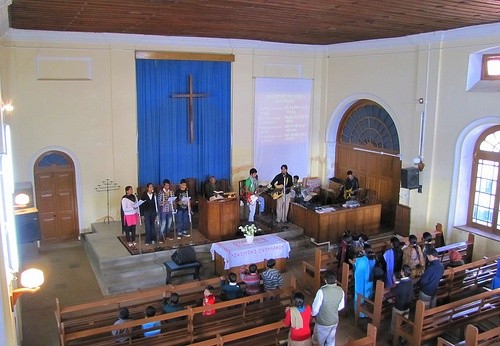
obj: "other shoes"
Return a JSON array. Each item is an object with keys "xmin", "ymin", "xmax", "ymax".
[
  {"xmin": 177, "ymin": 235, "xmax": 181, "ymax": 239},
  {"xmin": 127, "ymin": 242, "xmax": 133, "ymax": 246},
  {"xmin": 152, "ymin": 241, "xmax": 155, "ymax": 244},
  {"xmin": 166, "ymin": 236, "xmax": 174, "ymax": 240},
  {"xmin": 146, "ymin": 243, "xmax": 149, "ymax": 246},
  {"xmin": 159, "ymin": 239, "xmax": 163, "ymax": 243},
  {"xmin": 183, "ymin": 233, "xmax": 190, "ymax": 237},
  {"xmin": 132, "ymin": 241, "xmax": 137, "ymax": 245}
]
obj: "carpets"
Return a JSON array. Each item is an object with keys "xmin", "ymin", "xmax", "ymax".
[{"xmin": 118, "ymin": 217, "xmax": 284, "ymax": 255}]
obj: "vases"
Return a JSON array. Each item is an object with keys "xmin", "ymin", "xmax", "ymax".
[{"xmin": 245, "ymin": 235, "xmax": 254, "ymax": 243}]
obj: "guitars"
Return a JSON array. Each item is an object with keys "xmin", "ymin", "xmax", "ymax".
[
  {"xmin": 246, "ymin": 183, "xmax": 272, "ymax": 206},
  {"xmin": 344, "ymin": 188, "xmax": 363, "ymax": 200},
  {"xmin": 271, "ymin": 184, "xmax": 295, "ymax": 200}
]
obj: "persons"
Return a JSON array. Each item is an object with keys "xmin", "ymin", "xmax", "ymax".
[
  {"xmin": 245, "ymin": 168, "xmax": 264, "ymax": 225},
  {"xmin": 283, "ymin": 292, "xmax": 312, "ymax": 346},
  {"xmin": 220, "ymin": 272, "xmax": 244, "ymax": 310},
  {"xmin": 162, "ymin": 291, "xmax": 186, "ymax": 323},
  {"xmin": 312, "ymin": 270, "xmax": 345, "ymax": 346},
  {"xmin": 121, "ymin": 186, "xmax": 140, "ymax": 247},
  {"xmin": 446, "ymin": 249, "xmax": 465, "ymax": 284},
  {"xmin": 271, "ymin": 165, "xmax": 293, "ymax": 224},
  {"xmin": 340, "ymin": 170, "xmax": 359, "ymax": 203},
  {"xmin": 157, "ymin": 179, "xmax": 175, "ymax": 243},
  {"xmin": 293, "ymin": 175, "xmax": 299, "ymax": 187},
  {"xmin": 333, "ymin": 223, "xmax": 448, "ymax": 346},
  {"xmin": 174, "ymin": 179, "xmax": 190, "ymax": 240},
  {"xmin": 203, "ymin": 284, "xmax": 216, "ymax": 315},
  {"xmin": 138, "ymin": 183, "xmax": 158, "ymax": 245},
  {"xmin": 141, "ymin": 306, "xmax": 161, "ymax": 337},
  {"xmin": 111, "ymin": 307, "xmax": 135, "ymax": 342},
  {"xmin": 259, "ymin": 259, "xmax": 283, "ymax": 300},
  {"xmin": 240, "ymin": 263, "xmax": 260, "ymax": 295},
  {"xmin": 204, "ymin": 176, "xmax": 217, "ymax": 200}
]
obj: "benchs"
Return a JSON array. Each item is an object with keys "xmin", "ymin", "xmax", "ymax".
[{"xmin": 54, "ymin": 224, "xmax": 500, "ymax": 346}]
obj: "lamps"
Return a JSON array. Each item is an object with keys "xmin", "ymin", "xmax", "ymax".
[
  {"xmin": 413, "ymin": 157, "xmax": 424, "ymax": 171},
  {"xmin": 13, "ymin": 193, "xmax": 29, "ymax": 208},
  {"xmin": 9, "ymin": 268, "xmax": 44, "ymax": 312}
]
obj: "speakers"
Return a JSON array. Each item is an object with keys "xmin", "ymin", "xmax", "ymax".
[
  {"xmin": 171, "ymin": 246, "xmax": 196, "ymax": 265},
  {"xmin": 401, "ymin": 167, "xmax": 419, "ymax": 189}
]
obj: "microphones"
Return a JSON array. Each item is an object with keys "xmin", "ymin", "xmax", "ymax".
[
  {"xmin": 135, "ymin": 192, "xmax": 137, "ymax": 195},
  {"xmin": 186, "ymin": 186, "xmax": 188, "ymax": 191},
  {"xmin": 170, "ymin": 186, "xmax": 173, "ymax": 194},
  {"xmin": 151, "ymin": 190, "xmax": 156, "ymax": 194}
]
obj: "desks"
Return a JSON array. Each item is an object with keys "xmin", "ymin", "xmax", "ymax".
[
  {"xmin": 210, "ymin": 234, "xmax": 291, "ymax": 280},
  {"xmin": 197, "ymin": 197, "xmax": 240, "ymax": 242},
  {"xmin": 290, "ymin": 199, "xmax": 382, "ymax": 244},
  {"xmin": 163, "ymin": 260, "xmax": 201, "ymax": 284}
]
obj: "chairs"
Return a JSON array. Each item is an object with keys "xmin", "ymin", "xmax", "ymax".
[
  {"xmin": 238, "ymin": 179, "xmax": 259, "ymax": 220},
  {"xmin": 137, "ymin": 179, "xmax": 229, "ymax": 225}
]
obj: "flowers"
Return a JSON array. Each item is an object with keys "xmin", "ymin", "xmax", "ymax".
[{"xmin": 239, "ymin": 224, "xmax": 261, "ymax": 236}]
